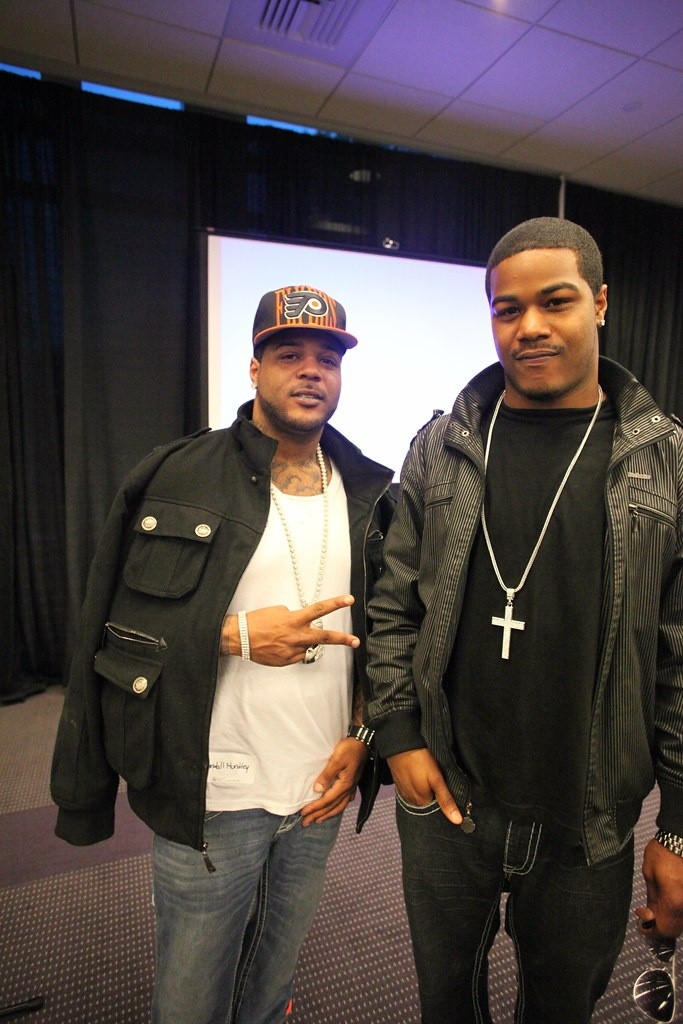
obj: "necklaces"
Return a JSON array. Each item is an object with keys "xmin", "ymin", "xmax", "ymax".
[
  {"xmin": 262, "ymin": 436, "xmax": 334, "ymax": 660},
  {"xmin": 473, "ymin": 385, "xmax": 603, "ymax": 663}
]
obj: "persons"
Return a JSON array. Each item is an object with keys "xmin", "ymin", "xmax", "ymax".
[
  {"xmin": 357, "ymin": 216, "xmax": 683, "ymax": 1024},
  {"xmin": 48, "ymin": 280, "xmax": 412, "ymax": 1024}
]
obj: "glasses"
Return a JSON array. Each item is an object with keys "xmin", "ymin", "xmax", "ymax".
[{"xmin": 633, "ymin": 938, "xmax": 675, "ymax": 1024}]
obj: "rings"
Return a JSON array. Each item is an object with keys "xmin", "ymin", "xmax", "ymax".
[{"xmin": 304, "ymin": 644, "xmax": 319, "ymax": 665}]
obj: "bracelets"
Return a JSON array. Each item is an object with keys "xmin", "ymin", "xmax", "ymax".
[
  {"xmin": 236, "ymin": 610, "xmax": 252, "ymax": 665},
  {"xmin": 656, "ymin": 828, "xmax": 683, "ymax": 859},
  {"xmin": 348, "ymin": 723, "xmax": 375, "ymax": 747}
]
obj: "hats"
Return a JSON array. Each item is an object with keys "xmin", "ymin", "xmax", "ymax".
[{"xmin": 251, "ymin": 285, "xmax": 358, "ymax": 349}]
